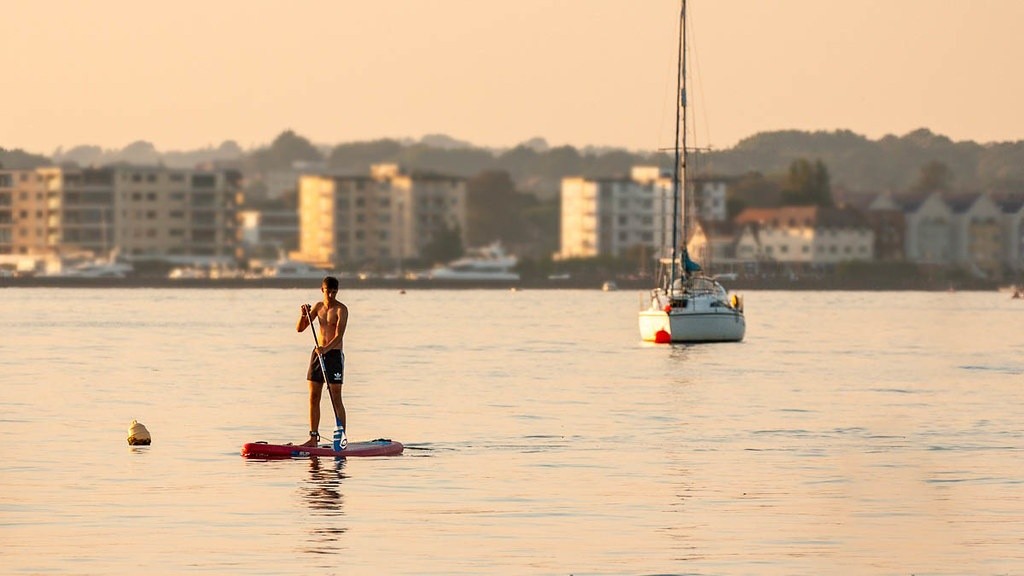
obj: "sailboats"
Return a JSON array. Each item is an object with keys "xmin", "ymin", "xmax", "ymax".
[{"xmin": 635, "ymin": 0, "xmax": 747, "ymax": 344}]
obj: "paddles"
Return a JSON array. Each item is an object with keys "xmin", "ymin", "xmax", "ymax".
[{"xmin": 304, "ymin": 304, "xmax": 348, "ymax": 452}]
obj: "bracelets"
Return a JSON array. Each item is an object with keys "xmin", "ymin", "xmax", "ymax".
[{"xmin": 301, "ymin": 314, "xmax": 307, "ymax": 317}]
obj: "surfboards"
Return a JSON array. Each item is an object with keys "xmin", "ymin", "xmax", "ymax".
[{"xmin": 240, "ymin": 438, "xmax": 404, "ymax": 457}]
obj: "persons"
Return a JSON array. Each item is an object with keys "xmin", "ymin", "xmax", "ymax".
[{"xmin": 296, "ymin": 276, "xmax": 348, "ymax": 446}]
